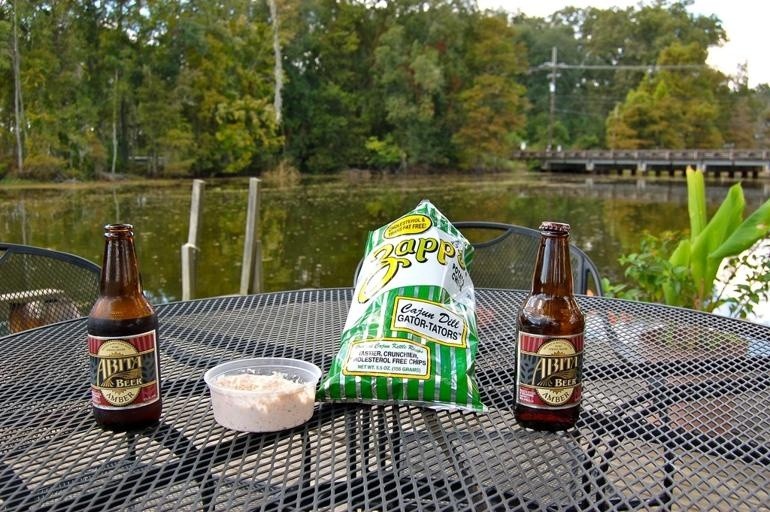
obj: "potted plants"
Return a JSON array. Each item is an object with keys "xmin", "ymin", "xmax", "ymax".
[{"xmin": 596, "ymin": 166, "xmax": 770, "ymax": 438}]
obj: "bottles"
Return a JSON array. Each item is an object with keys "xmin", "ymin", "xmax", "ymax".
[
  {"xmin": 514, "ymin": 221, "xmax": 584, "ymax": 434},
  {"xmin": 87, "ymin": 224, "xmax": 161, "ymax": 428}
]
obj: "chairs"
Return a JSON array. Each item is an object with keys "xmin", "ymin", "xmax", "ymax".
[
  {"xmin": 353, "ymin": 222, "xmax": 677, "ymax": 505},
  {"xmin": 0, "ymin": 242, "xmax": 313, "ymax": 512}
]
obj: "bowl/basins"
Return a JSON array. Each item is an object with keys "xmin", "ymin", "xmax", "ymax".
[{"xmin": 203, "ymin": 357, "xmax": 322, "ymax": 433}]
